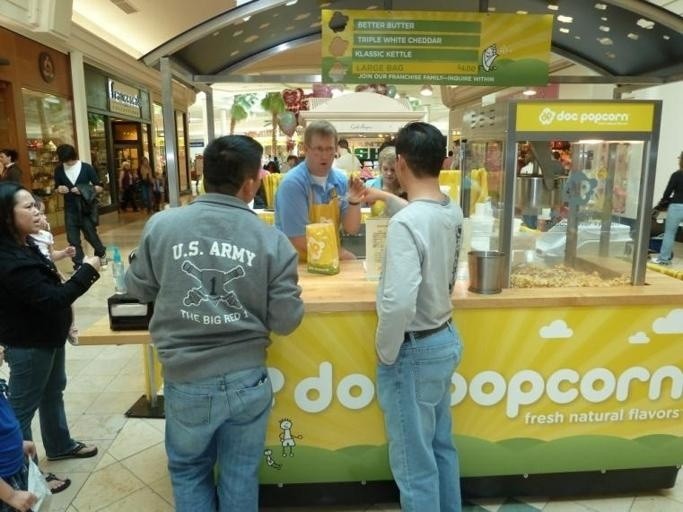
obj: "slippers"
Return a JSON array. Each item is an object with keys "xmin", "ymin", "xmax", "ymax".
[
  {"xmin": 47, "ymin": 441, "xmax": 98, "ymax": 462},
  {"xmin": 45, "ymin": 473, "xmax": 71, "ymax": 494}
]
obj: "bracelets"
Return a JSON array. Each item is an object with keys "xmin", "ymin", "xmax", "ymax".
[{"xmin": 349, "ymin": 201, "xmax": 359, "ymax": 206}]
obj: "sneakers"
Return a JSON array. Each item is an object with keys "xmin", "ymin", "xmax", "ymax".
[{"xmin": 100, "ymin": 254, "xmax": 109, "ymax": 270}]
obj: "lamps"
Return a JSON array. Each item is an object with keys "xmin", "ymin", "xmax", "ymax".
[{"xmin": 419, "ymin": 85, "xmax": 433, "ymax": 97}]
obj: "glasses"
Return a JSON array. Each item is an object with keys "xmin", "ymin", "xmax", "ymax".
[{"xmin": 305, "ymin": 143, "xmax": 335, "ymax": 156}]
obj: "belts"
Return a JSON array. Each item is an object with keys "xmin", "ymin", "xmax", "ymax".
[{"xmin": 404, "ymin": 316, "xmax": 452, "ymax": 342}]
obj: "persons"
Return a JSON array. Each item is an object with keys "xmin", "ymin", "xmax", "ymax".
[
  {"xmin": 125, "ymin": 132, "xmax": 304, "ymax": 512},
  {"xmin": 116, "ymin": 155, "xmax": 165, "ymax": 213},
  {"xmin": 366, "ymin": 139, "xmax": 491, "ymax": 213},
  {"xmin": 650, "ymin": 151, "xmax": 683, "ymax": 266},
  {"xmin": 1, "ymin": 338, "xmax": 40, "ymax": 511},
  {"xmin": 276, "ymin": 120, "xmax": 364, "ymax": 262},
  {"xmin": 519, "ymin": 150, "xmax": 570, "ymax": 179},
  {"xmin": 0, "ymin": 184, "xmax": 101, "ymax": 495},
  {"xmin": 52, "ymin": 146, "xmax": 107, "ymax": 277},
  {"xmin": 1, "ymin": 147, "xmax": 78, "ymax": 348},
  {"xmin": 254, "ymin": 140, "xmax": 356, "ymax": 192},
  {"xmin": 363, "ymin": 121, "xmax": 463, "ymax": 512}
]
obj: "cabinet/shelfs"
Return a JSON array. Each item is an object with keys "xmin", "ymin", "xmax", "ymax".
[{"xmin": 28, "ymin": 144, "xmax": 64, "ymax": 213}]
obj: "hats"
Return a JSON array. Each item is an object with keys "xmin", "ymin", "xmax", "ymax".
[{"xmin": 57, "ymin": 144, "xmax": 74, "ymax": 161}]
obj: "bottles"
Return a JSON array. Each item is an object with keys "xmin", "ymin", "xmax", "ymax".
[{"xmin": 111, "ymin": 246, "xmax": 127, "ymax": 294}]
